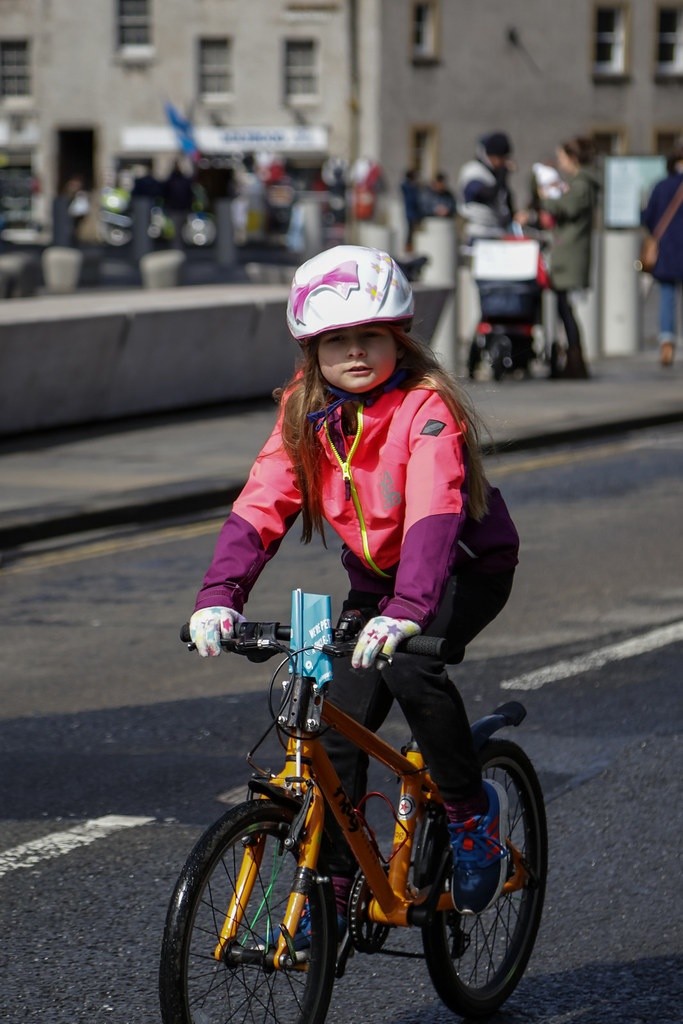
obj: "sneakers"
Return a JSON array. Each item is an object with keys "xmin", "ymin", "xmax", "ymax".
[
  {"xmin": 252, "ymin": 876, "xmax": 354, "ymax": 966},
  {"xmin": 447, "ymin": 778, "xmax": 509, "ymax": 916}
]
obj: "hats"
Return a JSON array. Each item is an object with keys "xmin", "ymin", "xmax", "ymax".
[{"xmin": 479, "ymin": 132, "xmax": 509, "ymax": 153}]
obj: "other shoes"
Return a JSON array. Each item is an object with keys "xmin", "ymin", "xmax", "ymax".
[{"xmin": 661, "ymin": 342, "xmax": 674, "ymax": 365}]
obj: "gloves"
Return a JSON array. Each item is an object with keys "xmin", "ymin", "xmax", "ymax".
[
  {"xmin": 351, "ymin": 615, "xmax": 422, "ymax": 671},
  {"xmin": 189, "ymin": 606, "xmax": 249, "ymax": 658}
]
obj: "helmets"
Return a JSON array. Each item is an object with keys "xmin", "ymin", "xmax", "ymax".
[{"xmin": 285, "ymin": 244, "xmax": 414, "ymax": 340}]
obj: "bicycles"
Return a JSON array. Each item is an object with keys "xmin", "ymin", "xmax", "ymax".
[{"xmin": 155, "ymin": 608, "xmax": 552, "ymax": 1024}]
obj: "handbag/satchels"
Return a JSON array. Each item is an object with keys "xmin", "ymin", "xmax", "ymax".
[{"xmin": 639, "ymin": 238, "xmax": 659, "ymax": 273}]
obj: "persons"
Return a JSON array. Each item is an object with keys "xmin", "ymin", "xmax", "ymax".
[
  {"xmin": 59, "ymin": 130, "xmax": 599, "ymax": 380},
  {"xmin": 645, "ymin": 153, "xmax": 683, "ymax": 364},
  {"xmin": 188, "ymin": 245, "xmax": 521, "ymax": 958}
]
